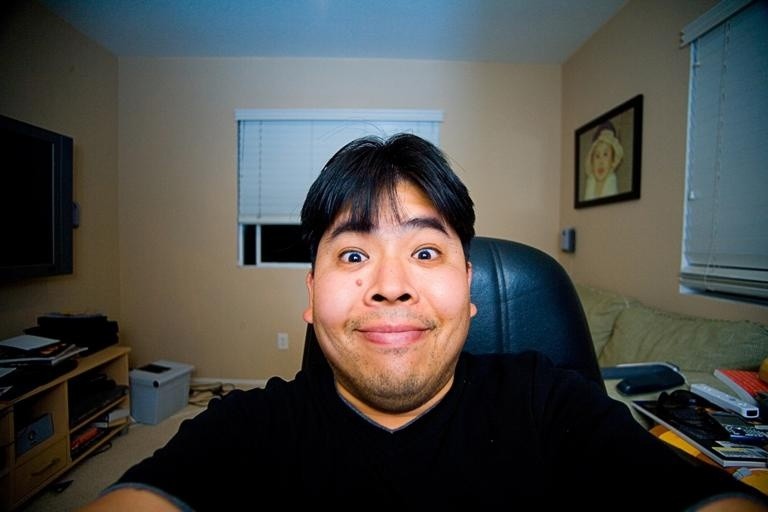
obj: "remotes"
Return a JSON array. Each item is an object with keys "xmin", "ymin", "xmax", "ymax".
[
  {"xmin": 615, "ymin": 370, "xmax": 684, "ymax": 396},
  {"xmin": 600, "ymin": 363, "xmax": 674, "ymax": 382}
]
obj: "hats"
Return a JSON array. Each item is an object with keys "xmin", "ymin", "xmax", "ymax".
[{"xmin": 584, "ymin": 125, "xmax": 622, "ymax": 176}]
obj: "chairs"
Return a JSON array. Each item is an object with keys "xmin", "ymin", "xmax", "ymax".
[{"xmin": 461, "ymin": 235, "xmax": 607, "ymax": 400}]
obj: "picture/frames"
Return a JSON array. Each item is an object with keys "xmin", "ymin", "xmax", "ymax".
[{"xmin": 574, "ymin": 93, "xmax": 644, "ymax": 211}]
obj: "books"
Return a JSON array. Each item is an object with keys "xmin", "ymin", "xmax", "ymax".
[
  {"xmin": 0, "ymin": 331, "xmax": 89, "ymax": 404},
  {"xmin": 629, "ymin": 358, "xmax": 767, "ymax": 473}
]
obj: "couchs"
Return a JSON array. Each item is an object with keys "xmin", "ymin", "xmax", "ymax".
[{"xmin": 572, "ymin": 282, "xmax": 768, "ymax": 432}]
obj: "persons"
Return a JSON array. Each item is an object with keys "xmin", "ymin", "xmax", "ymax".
[
  {"xmin": 581, "ymin": 119, "xmax": 625, "ymax": 203},
  {"xmin": 80, "ymin": 130, "xmax": 766, "ymax": 512}
]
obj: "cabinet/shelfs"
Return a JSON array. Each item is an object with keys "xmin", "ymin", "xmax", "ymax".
[{"xmin": 0, "ymin": 344, "xmax": 133, "ymax": 512}]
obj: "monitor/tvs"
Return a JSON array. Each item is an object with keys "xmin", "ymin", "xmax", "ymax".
[{"xmin": 0, "ymin": 114, "xmax": 73, "ymax": 289}]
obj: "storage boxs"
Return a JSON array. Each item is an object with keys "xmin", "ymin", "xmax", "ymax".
[{"xmin": 129, "ymin": 359, "xmax": 195, "ymax": 427}]
